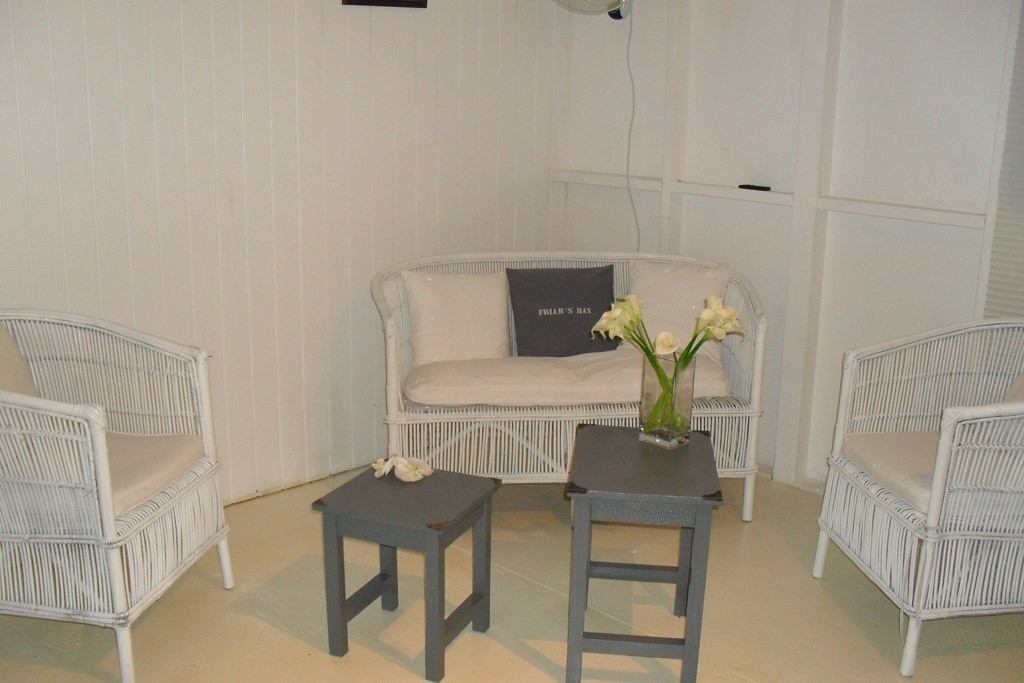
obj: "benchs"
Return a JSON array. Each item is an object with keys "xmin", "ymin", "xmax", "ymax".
[{"xmin": 369, "ymin": 251, "xmax": 767, "ymax": 522}]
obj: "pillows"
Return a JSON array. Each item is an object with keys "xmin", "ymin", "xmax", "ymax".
[
  {"xmin": 401, "ymin": 270, "xmax": 512, "ymax": 365},
  {"xmin": 505, "ymin": 264, "xmax": 622, "ymax": 358},
  {"xmin": 627, "ymin": 258, "xmax": 725, "ymax": 367}
]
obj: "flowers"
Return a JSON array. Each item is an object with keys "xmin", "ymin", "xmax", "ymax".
[
  {"xmin": 371, "ymin": 457, "xmax": 433, "ymax": 482},
  {"xmin": 589, "ymin": 294, "xmax": 746, "ymax": 429}
]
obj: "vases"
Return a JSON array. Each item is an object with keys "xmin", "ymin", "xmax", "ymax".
[{"xmin": 638, "ymin": 351, "xmax": 696, "ymax": 451}]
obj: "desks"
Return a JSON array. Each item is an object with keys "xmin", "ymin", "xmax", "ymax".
[
  {"xmin": 311, "ymin": 459, "xmax": 502, "ymax": 683},
  {"xmin": 565, "ymin": 425, "xmax": 723, "ymax": 683}
]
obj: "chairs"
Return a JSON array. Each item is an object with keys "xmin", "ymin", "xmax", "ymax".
[
  {"xmin": 813, "ymin": 319, "xmax": 1024, "ymax": 676},
  {"xmin": 0, "ymin": 308, "xmax": 236, "ymax": 683}
]
